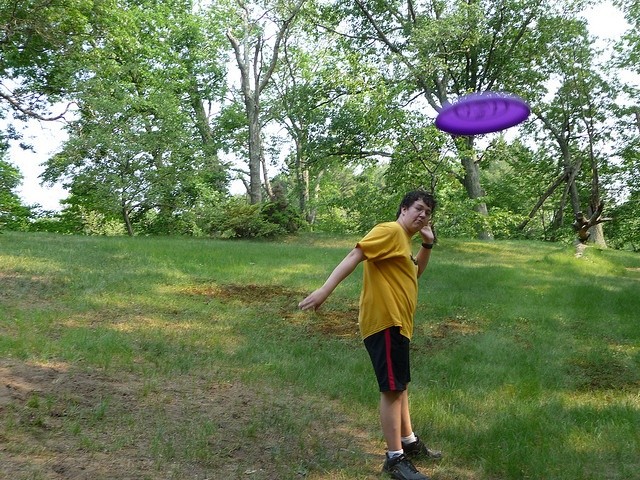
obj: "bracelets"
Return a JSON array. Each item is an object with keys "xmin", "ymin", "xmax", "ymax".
[{"xmin": 420, "ymin": 243, "xmax": 433, "ymax": 249}]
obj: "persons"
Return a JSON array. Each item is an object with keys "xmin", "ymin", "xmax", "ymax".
[{"xmin": 297, "ymin": 190, "xmax": 445, "ymax": 478}]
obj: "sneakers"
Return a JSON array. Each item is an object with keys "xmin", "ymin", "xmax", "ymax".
[
  {"xmin": 382, "ymin": 452, "xmax": 430, "ymax": 480},
  {"xmin": 401, "ymin": 435, "xmax": 442, "ymax": 461}
]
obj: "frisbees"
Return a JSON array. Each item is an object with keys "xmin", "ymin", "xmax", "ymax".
[{"xmin": 435, "ymin": 93, "xmax": 531, "ymax": 135}]
